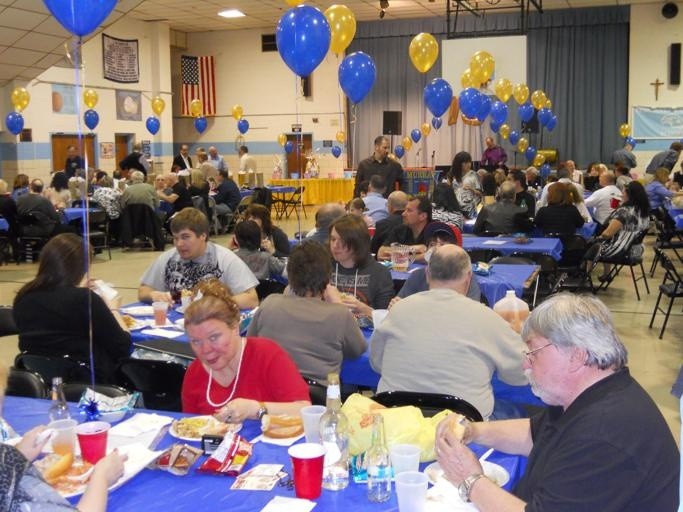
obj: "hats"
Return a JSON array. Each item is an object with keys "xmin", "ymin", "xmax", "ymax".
[{"xmin": 422, "ymin": 220, "xmax": 462, "ymax": 249}]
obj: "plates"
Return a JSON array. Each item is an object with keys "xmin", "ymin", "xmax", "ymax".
[
  {"xmin": 119, "ymin": 305, "xmax": 187, "ymax": 332},
  {"xmin": 65, "ymin": 464, "xmax": 93, "ymax": 482},
  {"xmin": 80, "ymin": 411, "xmax": 126, "ymax": 422},
  {"xmin": 169, "ymin": 414, "xmax": 242, "ymax": 442},
  {"xmin": 424, "ymin": 461, "xmax": 511, "ymax": 492}
]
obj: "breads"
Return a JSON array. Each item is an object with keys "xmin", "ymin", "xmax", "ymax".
[{"xmin": 37, "ymin": 453, "xmax": 92, "ymax": 488}]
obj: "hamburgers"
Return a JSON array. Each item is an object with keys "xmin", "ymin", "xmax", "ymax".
[
  {"xmin": 436, "ymin": 414, "xmax": 471, "ymax": 444},
  {"xmin": 261, "ymin": 413, "xmax": 303, "ymax": 437}
]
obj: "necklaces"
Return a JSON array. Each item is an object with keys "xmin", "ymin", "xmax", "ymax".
[{"xmin": 203, "ymin": 340, "xmax": 248, "ymax": 407}]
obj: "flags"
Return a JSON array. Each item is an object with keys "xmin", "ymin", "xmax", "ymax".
[{"xmin": 180, "ymin": 55, "xmax": 216, "ymax": 115}]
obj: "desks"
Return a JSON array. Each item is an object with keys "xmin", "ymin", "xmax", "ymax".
[{"xmin": 268, "ymin": 177, "xmax": 355, "ymax": 206}]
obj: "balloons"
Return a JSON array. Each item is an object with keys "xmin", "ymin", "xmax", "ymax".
[
  {"xmin": 231, "ymin": 104, "xmax": 243, "ymax": 124},
  {"xmin": 237, "ymin": 120, "xmax": 248, "ymax": 135},
  {"xmin": 190, "ymin": 99, "xmax": 202, "ymax": 117},
  {"xmin": 618, "ymin": 124, "xmax": 631, "ymax": 138},
  {"xmin": 144, "ymin": 116, "xmax": 160, "ymax": 136},
  {"xmin": 149, "ymin": 96, "xmax": 166, "ymax": 116},
  {"xmin": 82, "ymin": 89, "xmax": 98, "ymax": 109},
  {"xmin": 194, "ymin": 116, "xmax": 208, "ymax": 134},
  {"xmin": 275, "ymin": 5, "xmax": 330, "ymax": 81},
  {"xmin": 42, "ymin": 0, "xmax": 120, "ymax": 37},
  {"xmin": 338, "ymin": 52, "xmax": 377, "ymax": 107},
  {"xmin": 323, "ymin": 2, "xmax": 358, "ymax": 54},
  {"xmin": 407, "ymin": 33, "xmax": 558, "ymax": 134},
  {"xmin": 336, "ymin": 132, "xmax": 345, "ymax": 144},
  {"xmin": 4, "ymin": 112, "xmax": 25, "ymax": 137},
  {"xmin": 284, "ymin": 1, "xmax": 304, "ymax": 7},
  {"xmin": 82, "ymin": 110, "xmax": 99, "ymax": 133},
  {"xmin": 10, "ymin": 86, "xmax": 31, "ymax": 113}
]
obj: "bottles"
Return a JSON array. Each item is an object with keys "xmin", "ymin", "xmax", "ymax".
[
  {"xmin": 49, "ymin": 379, "xmax": 71, "ymax": 424},
  {"xmin": 315, "ymin": 373, "xmax": 350, "ymax": 492},
  {"xmin": 366, "ymin": 417, "xmax": 392, "ymax": 502}
]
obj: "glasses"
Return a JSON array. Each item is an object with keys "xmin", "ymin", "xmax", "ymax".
[{"xmin": 520, "ymin": 341, "xmax": 555, "ymax": 364}]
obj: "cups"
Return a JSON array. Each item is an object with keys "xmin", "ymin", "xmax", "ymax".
[
  {"xmin": 389, "ymin": 244, "xmax": 415, "ymax": 272},
  {"xmin": 152, "ymin": 301, "xmax": 167, "ymax": 327},
  {"xmin": 392, "ymin": 444, "xmax": 422, "ymax": 471},
  {"xmin": 300, "ymin": 405, "xmax": 327, "ymax": 445},
  {"xmin": 76, "ymin": 421, "xmax": 111, "ymax": 462},
  {"xmin": 43, "ymin": 420, "xmax": 78, "ymax": 461},
  {"xmin": 287, "ymin": 442, "xmax": 325, "ymax": 499},
  {"xmin": 394, "ymin": 472, "xmax": 430, "ymax": 512}
]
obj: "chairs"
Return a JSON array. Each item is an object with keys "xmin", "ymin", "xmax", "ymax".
[{"xmin": 584, "ymin": 203, "xmax": 683, "ymax": 340}]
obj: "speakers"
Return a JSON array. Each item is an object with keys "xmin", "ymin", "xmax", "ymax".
[
  {"xmin": 300, "ymin": 75, "xmax": 312, "ymax": 97},
  {"xmin": 521, "ymin": 108, "xmax": 538, "ymax": 134},
  {"xmin": 661, "ymin": 3, "xmax": 678, "ymax": 18},
  {"xmin": 670, "ymin": 43, "xmax": 681, "ymax": 85},
  {"xmin": 261, "ymin": 34, "xmax": 277, "ymax": 52},
  {"xmin": 382, "ymin": 110, "xmax": 402, "ymax": 135}
]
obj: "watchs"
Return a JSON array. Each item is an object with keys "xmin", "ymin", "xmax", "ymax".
[
  {"xmin": 457, "ymin": 474, "xmax": 484, "ymax": 504},
  {"xmin": 256, "ymin": 402, "xmax": 268, "ymax": 421}
]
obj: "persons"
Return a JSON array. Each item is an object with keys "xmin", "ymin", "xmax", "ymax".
[
  {"xmin": 13, "ymin": 229, "xmax": 131, "ymax": 393},
  {"xmin": 434, "ymin": 291, "xmax": 683, "ymax": 511},
  {"xmin": 0, "ymin": 425, "xmax": 130, "ymax": 512},
  {"xmin": 228, "ymin": 186, "xmax": 394, "ymax": 309},
  {"xmin": 139, "ymin": 208, "xmax": 258, "ymax": 309},
  {"xmin": 447, "ymin": 134, "xmax": 651, "ymax": 290},
  {"xmin": 1, "ymin": 132, "xmax": 258, "ymax": 265},
  {"xmin": 244, "ymin": 244, "xmax": 365, "ymax": 407},
  {"xmin": 180, "ymin": 277, "xmax": 312, "ymax": 422},
  {"xmin": 369, "ymin": 244, "xmax": 530, "ymax": 423},
  {"xmin": 353, "ymin": 135, "xmax": 456, "ymax": 246},
  {"xmin": 646, "ymin": 141, "xmax": 683, "ymax": 248}
]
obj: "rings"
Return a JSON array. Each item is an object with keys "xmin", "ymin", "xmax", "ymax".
[
  {"xmin": 436, "ymin": 448, "xmax": 440, "ymax": 453},
  {"xmin": 224, "ymin": 415, "xmax": 232, "ymax": 422}
]
